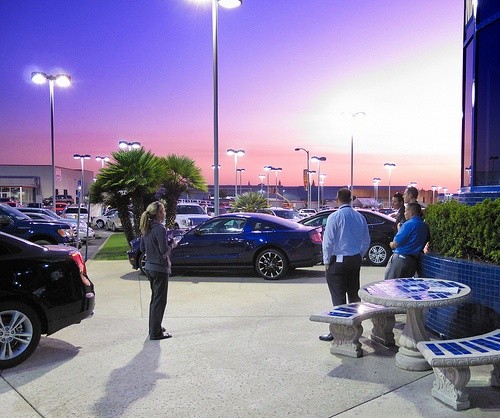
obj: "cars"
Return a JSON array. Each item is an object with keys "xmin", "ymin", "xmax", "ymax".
[
  {"xmin": 295, "ymin": 209, "xmax": 397, "ymax": 268},
  {"xmin": 0, "ymin": 202, "xmax": 83, "ymax": 250},
  {"xmin": 126, "ymin": 213, "xmax": 324, "ymax": 281},
  {"xmin": 1, "ymin": 192, "xmax": 134, "ymax": 245},
  {"xmin": 171, "ymin": 199, "xmax": 317, "ymax": 231},
  {"xmin": 0, "ymin": 232, "xmax": 96, "ymax": 369},
  {"xmin": 352, "ymin": 197, "xmax": 428, "ymax": 220},
  {"xmin": 258, "ymin": 191, "xmax": 292, "ymax": 208}
]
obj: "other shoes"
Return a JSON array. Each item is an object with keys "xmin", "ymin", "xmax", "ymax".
[
  {"xmin": 319, "ymin": 333, "xmax": 335, "ymax": 341},
  {"xmin": 162, "ymin": 327, "xmax": 166, "ymax": 332},
  {"xmin": 164, "ymin": 332, "xmax": 172, "ymax": 338}
]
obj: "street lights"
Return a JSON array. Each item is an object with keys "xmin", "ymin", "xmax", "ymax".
[
  {"xmin": 226, "ymin": 148, "xmax": 245, "ymax": 199},
  {"xmin": 95, "ymin": 156, "xmax": 110, "ymax": 173},
  {"xmin": 29, "ymin": 70, "xmax": 73, "ymax": 216},
  {"xmin": 194, "ymin": 0, "xmax": 241, "ymax": 220},
  {"xmin": 306, "ymin": 170, "xmax": 316, "ymax": 208},
  {"xmin": 311, "ymin": 155, "xmax": 326, "ymax": 211},
  {"xmin": 384, "ymin": 163, "xmax": 396, "ymax": 208},
  {"xmin": 295, "ymin": 147, "xmax": 310, "ymax": 209},
  {"xmin": 236, "ymin": 168, "xmax": 245, "ymax": 197},
  {"xmin": 372, "ymin": 178, "xmax": 382, "ymax": 210},
  {"xmin": 340, "ymin": 110, "xmax": 366, "ymax": 208},
  {"xmin": 263, "ymin": 166, "xmax": 273, "ymax": 206},
  {"xmin": 270, "ymin": 168, "xmax": 283, "ymax": 209},
  {"xmin": 258, "ymin": 175, "xmax": 266, "ymax": 196},
  {"xmin": 73, "ymin": 153, "xmax": 91, "ymax": 250},
  {"xmin": 118, "ymin": 141, "xmax": 142, "ymax": 152},
  {"xmin": 442, "ymin": 187, "xmax": 447, "ymax": 202},
  {"xmin": 431, "ymin": 185, "xmax": 437, "ymax": 206}
]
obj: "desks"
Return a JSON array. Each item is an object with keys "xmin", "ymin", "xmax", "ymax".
[{"xmin": 358, "ymin": 277, "xmax": 472, "ymax": 372}]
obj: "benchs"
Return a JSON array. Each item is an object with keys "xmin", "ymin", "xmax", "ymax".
[
  {"xmin": 309, "ymin": 300, "xmax": 408, "ymax": 358},
  {"xmin": 416, "ymin": 328, "xmax": 500, "ymax": 411}
]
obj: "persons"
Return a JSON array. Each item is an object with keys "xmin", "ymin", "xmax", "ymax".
[
  {"xmin": 319, "ymin": 188, "xmax": 371, "ymax": 342},
  {"xmin": 392, "ymin": 186, "xmax": 424, "ymax": 237},
  {"xmin": 139, "ymin": 201, "xmax": 178, "ymax": 340},
  {"xmin": 384, "ymin": 203, "xmax": 430, "ymax": 280}
]
